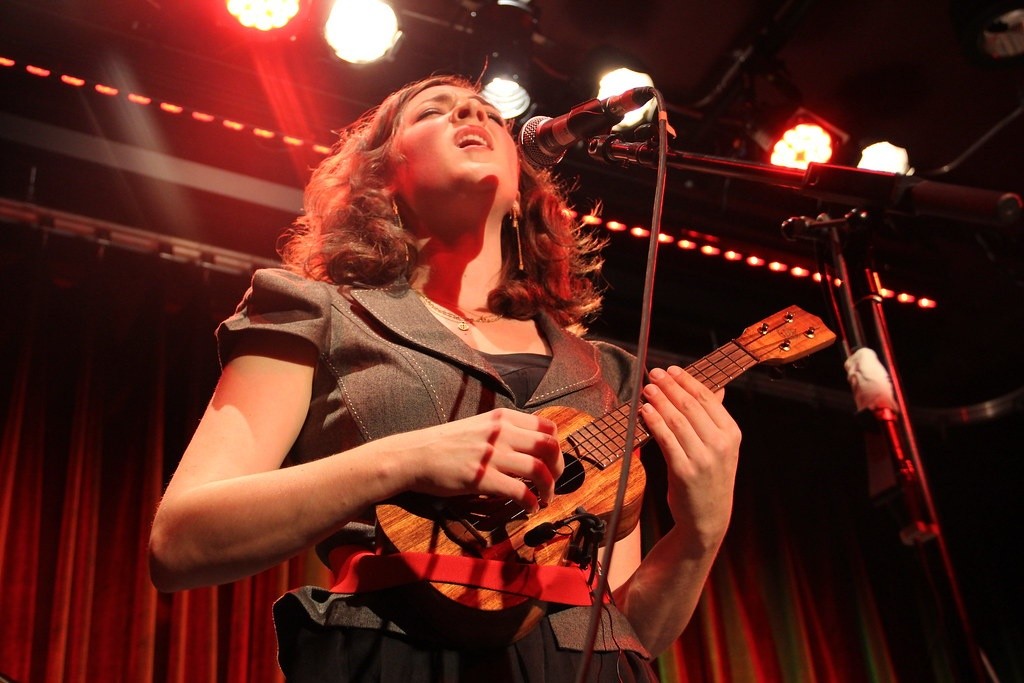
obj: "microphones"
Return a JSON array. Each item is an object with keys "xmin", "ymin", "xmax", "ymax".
[
  {"xmin": 519, "ymin": 86, "xmax": 656, "ymax": 169},
  {"xmin": 522, "ymin": 513, "xmax": 599, "ymax": 585}
]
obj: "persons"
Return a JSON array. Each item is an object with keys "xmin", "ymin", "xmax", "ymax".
[{"xmin": 149, "ymin": 77, "xmax": 741, "ymax": 683}]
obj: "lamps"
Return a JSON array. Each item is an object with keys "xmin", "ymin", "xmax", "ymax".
[
  {"xmin": 479, "ymin": 0, "xmax": 535, "ymax": 118},
  {"xmin": 585, "ymin": 44, "xmax": 659, "ymax": 129}
]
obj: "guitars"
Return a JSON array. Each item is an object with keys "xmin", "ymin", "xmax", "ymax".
[{"xmin": 371, "ymin": 301, "xmax": 837, "ymax": 651}]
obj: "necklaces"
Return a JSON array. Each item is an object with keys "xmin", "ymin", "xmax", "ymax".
[{"xmin": 411, "ymin": 286, "xmax": 506, "ymax": 332}]
